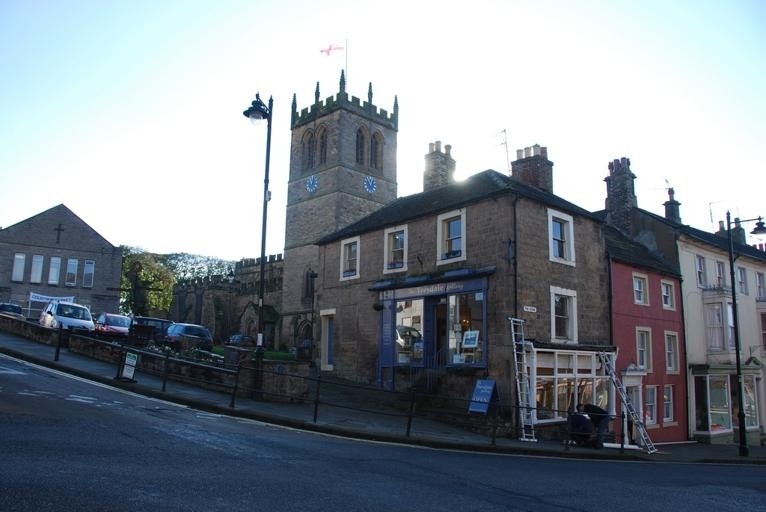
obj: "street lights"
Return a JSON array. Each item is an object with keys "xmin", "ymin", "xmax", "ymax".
[
  {"xmin": 243, "ymin": 93, "xmax": 273, "ymax": 400},
  {"xmin": 728, "ymin": 212, "xmax": 766, "ymax": 456}
]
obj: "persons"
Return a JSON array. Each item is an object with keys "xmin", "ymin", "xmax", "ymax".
[{"xmin": 570, "ymin": 403, "xmax": 609, "ymax": 449}]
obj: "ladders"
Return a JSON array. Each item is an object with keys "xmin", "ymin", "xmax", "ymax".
[
  {"xmin": 598, "ymin": 350, "xmax": 658, "ymax": 454},
  {"xmin": 508, "ymin": 317, "xmax": 539, "ymax": 443}
]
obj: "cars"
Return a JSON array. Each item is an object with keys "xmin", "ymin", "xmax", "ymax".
[
  {"xmin": 224, "ymin": 335, "xmax": 256, "ymax": 348},
  {"xmin": 0, "ymin": 299, "xmax": 214, "ymax": 354}
]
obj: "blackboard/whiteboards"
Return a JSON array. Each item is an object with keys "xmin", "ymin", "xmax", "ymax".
[{"xmin": 461, "ymin": 330, "xmax": 480, "ymax": 348}]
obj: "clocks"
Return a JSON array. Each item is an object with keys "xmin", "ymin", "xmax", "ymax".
[
  {"xmin": 364, "ymin": 176, "xmax": 377, "ymax": 193},
  {"xmin": 306, "ymin": 176, "xmax": 318, "ymax": 192}
]
